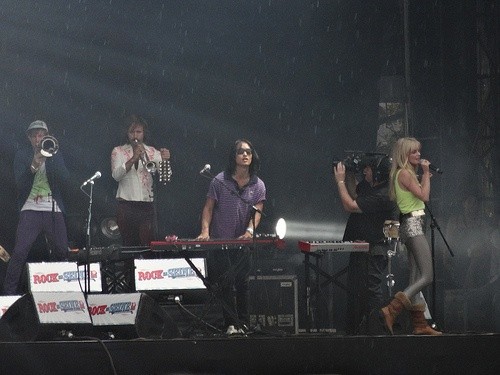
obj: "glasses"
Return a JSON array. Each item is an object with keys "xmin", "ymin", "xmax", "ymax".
[{"xmin": 236, "ymin": 148, "xmax": 253, "ymax": 154}]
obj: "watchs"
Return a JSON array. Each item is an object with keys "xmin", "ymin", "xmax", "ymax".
[{"xmin": 246, "ymin": 227, "xmax": 253, "ymax": 235}]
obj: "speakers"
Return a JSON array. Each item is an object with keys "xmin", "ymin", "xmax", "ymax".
[
  {"xmin": 0, "ymin": 292, "xmax": 40, "ymax": 341},
  {"xmin": 80, "ymin": 292, "xmax": 182, "ymax": 339}
]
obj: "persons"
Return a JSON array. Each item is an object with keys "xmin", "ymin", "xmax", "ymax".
[
  {"xmin": 333, "ymin": 137, "xmax": 442, "ymax": 334},
  {"xmin": 4, "ymin": 121, "xmax": 72, "ymax": 322},
  {"xmin": 111, "ymin": 116, "xmax": 171, "ymax": 246},
  {"xmin": 193, "ymin": 139, "xmax": 266, "ymax": 334}
]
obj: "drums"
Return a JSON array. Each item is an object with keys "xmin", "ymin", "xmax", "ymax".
[{"xmin": 383, "ymin": 220, "xmax": 401, "ymax": 241}]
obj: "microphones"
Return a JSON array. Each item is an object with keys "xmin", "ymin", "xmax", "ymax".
[
  {"xmin": 418, "ymin": 159, "xmax": 441, "ymax": 173},
  {"xmin": 200, "ymin": 164, "xmax": 211, "ymax": 173},
  {"xmin": 82, "ymin": 171, "xmax": 101, "ymax": 187},
  {"xmin": 49, "ymin": 148, "xmax": 56, "ymax": 156}
]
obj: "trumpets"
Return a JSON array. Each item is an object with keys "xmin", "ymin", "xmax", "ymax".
[{"xmin": 135, "ymin": 140, "xmax": 156, "ymax": 173}]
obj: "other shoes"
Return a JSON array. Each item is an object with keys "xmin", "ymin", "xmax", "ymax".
[{"xmin": 227, "ymin": 325, "xmax": 248, "ymax": 337}]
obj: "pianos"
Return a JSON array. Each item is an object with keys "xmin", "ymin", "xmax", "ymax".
[
  {"xmin": 299, "ymin": 239, "xmax": 369, "ymax": 326},
  {"xmin": 150, "ymin": 235, "xmax": 287, "ymax": 335}
]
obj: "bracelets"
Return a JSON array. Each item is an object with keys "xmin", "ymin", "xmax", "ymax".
[{"xmin": 338, "ymin": 181, "xmax": 344, "ymax": 184}]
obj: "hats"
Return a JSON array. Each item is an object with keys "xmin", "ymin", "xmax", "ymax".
[{"xmin": 28, "ymin": 120, "xmax": 48, "ymax": 133}]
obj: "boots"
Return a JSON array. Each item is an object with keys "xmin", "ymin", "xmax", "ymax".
[
  {"xmin": 377, "ymin": 291, "xmax": 412, "ymax": 336},
  {"xmin": 410, "ymin": 304, "xmax": 443, "ymax": 335}
]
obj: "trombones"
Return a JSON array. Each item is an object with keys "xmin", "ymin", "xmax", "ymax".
[{"xmin": 30, "ymin": 135, "xmax": 60, "ymax": 173}]
